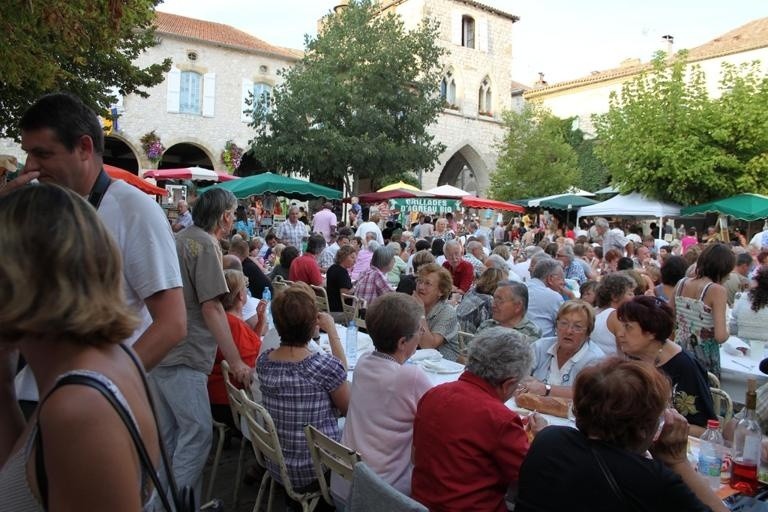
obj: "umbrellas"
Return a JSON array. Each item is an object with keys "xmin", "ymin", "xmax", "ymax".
[
  {"xmin": 143, "ymin": 167, "xmax": 240, "ymax": 182},
  {"xmin": 342, "ymin": 180, "xmax": 624, "ymax": 213},
  {"xmin": 680, "ymin": 191, "xmax": 768, "ymax": 222},
  {"xmin": 194, "ymin": 171, "xmax": 343, "ymax": 201},
  {"xmin": 103, "ymin": 163, "xmax": 169, "ymax": 196}
]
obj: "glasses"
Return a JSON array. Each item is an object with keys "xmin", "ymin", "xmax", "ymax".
[
  {"xmin": 557, "ymin": 254, "xmax": 568, "ymax": 257},
  {"xmin": 557, "ymin": 320, "xmax": 588, "ymax": 334},
  {"xmin": 412, "ymin": 327, "xmax": 426, "ymax": 336},
  {"xmin": 415, "ymin": 278, "xmax": 440, "ymax": 288},
  {"xmin": 242, "ymin": 276, "xmax": 249, "ymax": 288},
  {"xmin": 493, "ymin": 298, "xmax": 508, "ymax": 304}
]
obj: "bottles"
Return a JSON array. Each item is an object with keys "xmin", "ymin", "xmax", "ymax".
[
  {"xmin": 262, "ymin": 287, "xmax": 272, "ymax": 321},
  {"xmin": 696, "ymin": 420, "xmax": 724, "ymax": 491},
  {"xmin": 687, "ymin": 439, "xmax": 697, "ymax": 471},
  {"xmin": 729, "ymin": 378, "xmax": 763, "ymax": 497},
  {"xmin": 345, "ymin": 320, "xmax": 357, "ymax": 364}
]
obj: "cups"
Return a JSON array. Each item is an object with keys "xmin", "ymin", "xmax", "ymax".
[{"xmin": 750, "ymin": 340, "xmax": 763, "ymax": 362}]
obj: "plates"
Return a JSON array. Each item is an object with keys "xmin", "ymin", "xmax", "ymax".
[{"xmin": 422, "ymin": 359, "xmax": 465, "ymax": 374}]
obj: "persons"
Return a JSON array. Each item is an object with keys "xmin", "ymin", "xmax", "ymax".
[
  {"xmin": 147, "ymin": 187, "xmax": 253, "ymax": 512},
  {"xmin": 0, "ymin": 182, "xmax": 177, "ymax": 512},
  {"xmin": 172, "ymin": 198, "xmax": 767, "ymax": 512},
  {"xmin": 0, "ymin": 94, "xmax": 188, "ymax": 372}
]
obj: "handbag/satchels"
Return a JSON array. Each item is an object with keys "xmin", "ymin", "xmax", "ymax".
[{"xmin": 723, "ymin": 492, "xmax": 768, "ymax": 512}]
